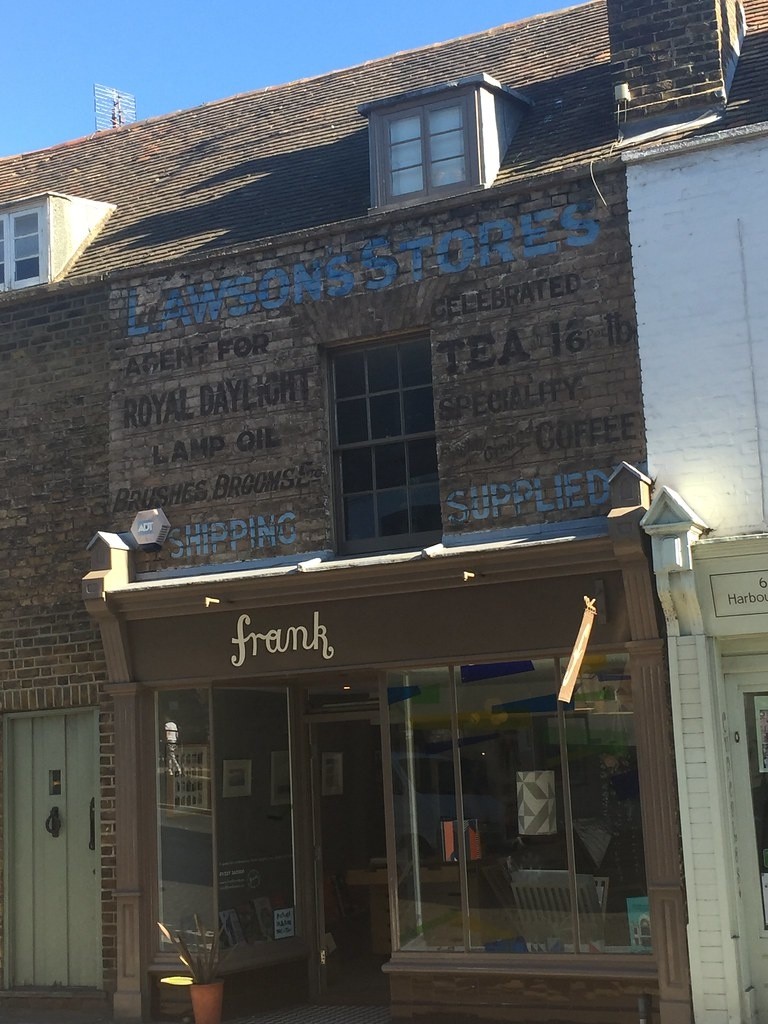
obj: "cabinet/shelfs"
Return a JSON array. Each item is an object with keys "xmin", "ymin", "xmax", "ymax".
[{"xmin": 348, "ymin": 863, "xmax": 521, "ymax": 952}]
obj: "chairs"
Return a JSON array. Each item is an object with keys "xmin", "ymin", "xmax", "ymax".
[{"xmin": 508, "ymin": 873, "xmax": 610, "ymax": 954}]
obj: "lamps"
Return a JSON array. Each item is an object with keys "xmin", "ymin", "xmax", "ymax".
[{"xmin": 515, "ymin": 715, "xmax": 559, "ymax": 836}]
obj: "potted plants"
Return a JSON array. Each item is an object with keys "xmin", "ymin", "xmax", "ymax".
[{"xmin": 156, "ymin": 913, "xmax": 239, "ymax": 1024}]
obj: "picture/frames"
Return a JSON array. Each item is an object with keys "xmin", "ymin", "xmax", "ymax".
[
  {"xmin": 165, "ymin": 744, "xmax": 211, "ymax": 817},
  {"xmin": 271, "ymin": 750, "xmax": 292, "ymax": 805},
  {"xmin": 222, "ymin": 759, "xmax": 252, "ymax": 798},
  {"xmin": 322, "ymin": 752, "xmax": 343, "ymax": 797}
]
